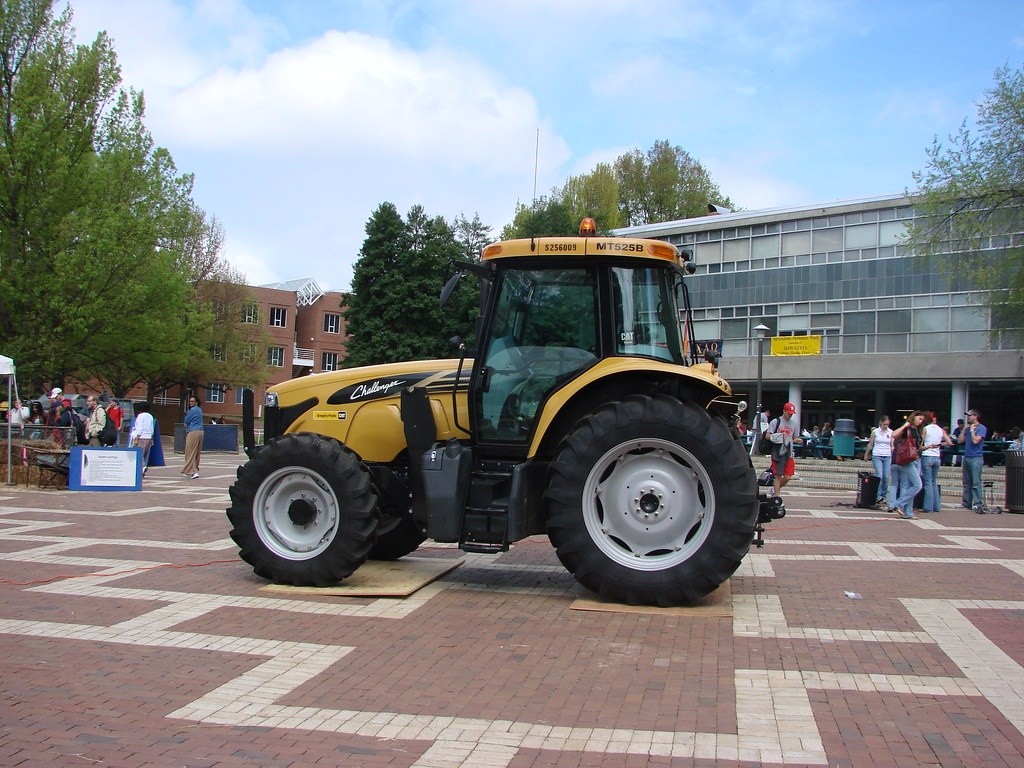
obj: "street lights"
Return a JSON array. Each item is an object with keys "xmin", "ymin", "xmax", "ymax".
[{"xmin": 750, "ymin": 323, "xmax": 771, "ymax": 455}]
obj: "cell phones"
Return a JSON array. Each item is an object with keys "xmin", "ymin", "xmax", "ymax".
[{"xmin": 936, "ymin": 443, "xmax": 941, "ymax": 446}]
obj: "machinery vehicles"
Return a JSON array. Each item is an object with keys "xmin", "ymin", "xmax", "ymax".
[{"xmin": 224, "ymin": 217, "xmax": 786, "ymax": 606}]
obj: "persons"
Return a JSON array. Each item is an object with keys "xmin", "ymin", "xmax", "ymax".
[
  {"xmin": 940, "ymin": 426, "xmax": 957, "ymax": 466},
  {"xmin": 765, "ymin": 403, "xmax": 803, "ymax": 495},
  {"xmin": 871, "ymin": 427, "xmax": 876, "ymax": 431},
  {"xmin": 6, "ymin": 387, "xmax": 93, "ymax": 448},
  {"xmin": 87, "ymin": 394, "xmax": 106, "ymax": 447},
  {"xmin": 990, "ymin": 427, "xmax": 1024, "ymax": 467},
  {"xmin": 886, "ymin": 410, "xmax": 951, "ymax": 519},
  {"xmin": 696, "ymin": 343, "xmax": 702, "ymax": 353},
  {"xmin": 130, "ymin": 401, "xmax": 154, "ymax": 476},
  {"xmin": 955, "ymin": 409, "xmax": 987, "ymax": 511},
  {"xmin": 735, "ymin": 420, "xmax": 748, "ymax": 444},
  {"xmin": 864, "ymin": 416, "xmax": 894, "ymax": 503},
  {"xmin": 703, "ymin": 343, "xmax": 709, "ymax": 353},
  {"xmin": 219, "ymin": 415, "xmax": 227, "ymax": 424},
  {"xmin": 104, "ymin": 398, "xmax": 124, "ymax": 446},
  {"xmin": 952, "ymin": 419, "xmax": 966, "ymax": 467},
  {"xmin": 181, "ymin": 396, "xmax": 204, "ymax": 479},
  {"xmin": 209, "ymin": 417, "xmax": 218, "ymax": 424},
  {"xmin": 801, "ymin": 422, "xmax": 872, "ymax": 462},
  {"xmin": 752, "ymin": 410, "xmax": 772, "ymax": 454}
]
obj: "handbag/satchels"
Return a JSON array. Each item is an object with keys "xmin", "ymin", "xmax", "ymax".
[
  {"xmin": 759, "ymin": 418, "xmax": 780, "ymax": 454},
  {"xmin": 96, "ymin": 407, "xmax": 117, "ymax": 445},
  {"xmin": 895, "ymin": 427, "xmax": 919, "ymax": 465}
]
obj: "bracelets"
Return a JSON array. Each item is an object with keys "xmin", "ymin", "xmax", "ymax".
[{"xmin": 969, "ymin": 430, "xmax": 976, "ymax": 433}]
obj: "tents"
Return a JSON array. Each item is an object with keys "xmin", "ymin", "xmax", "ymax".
[{"xmin": 0, "ymin": 355, "xmax": 25, "ymax": 484}]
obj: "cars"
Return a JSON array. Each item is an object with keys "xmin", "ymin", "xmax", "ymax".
[{"xmin": 1, "ymin": 392, "xmax": 105, "ymax": 471}]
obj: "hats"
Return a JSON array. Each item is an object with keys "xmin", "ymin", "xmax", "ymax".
[
  {"xmin": 964, "ymin": 409, "xmax": 981, "ymax": 418},
  {"xmin": 784, "ymin": 403, "xmax": 796, "ymax": 414},
  {"xmin": 51, "ymin": 400, "xmax": 63, "ymax": 410},
  {"xmin": 50, "ymin": 388, "xmax": 62, "ymax": 399}
]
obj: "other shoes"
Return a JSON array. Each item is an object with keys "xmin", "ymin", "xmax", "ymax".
[
  {"xmin": 972, "ymin": 506, "xmax": 978, "ymax": 510},
  {"xmin": 887, "ymin": 507, "xmax": 893, "ymax": 512},
  {"xmin": 191, "ymin": 473, "xmax": 199, "ymax": 479},
  {"xmin": 892, "ymin": 505, "xmax": 904, "ymax": 515},
  {"xmin": 956, "ymin": 505, "xmax": 967, "ymax": 509},
  {"xmin": 143, "ymin": 467, "xmax": 147, "ymax": 476},
  {"xmin": 911, "ymin": 517, "xmax": 918, "ymax": 519},
  {"xmin": 919, "ymin": 509, "xmax": 930, "ymax": 513}
]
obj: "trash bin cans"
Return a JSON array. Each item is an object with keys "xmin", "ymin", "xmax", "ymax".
[
  {"xmin": 1001, "ymin": 449, "xmax": 1024, "ymax": 514},
  {"xmin": 832, "ymin": 419, "xmax": 856, "ymax": 456}
]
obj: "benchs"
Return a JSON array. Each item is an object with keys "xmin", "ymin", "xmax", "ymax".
[
  {"xmin": 940, "ymin": 448, "xmax": 997, "ymax": 466},
  {"xmin": 793, "ymin": 443, "xmax": 874, "ymax": 454}
]
